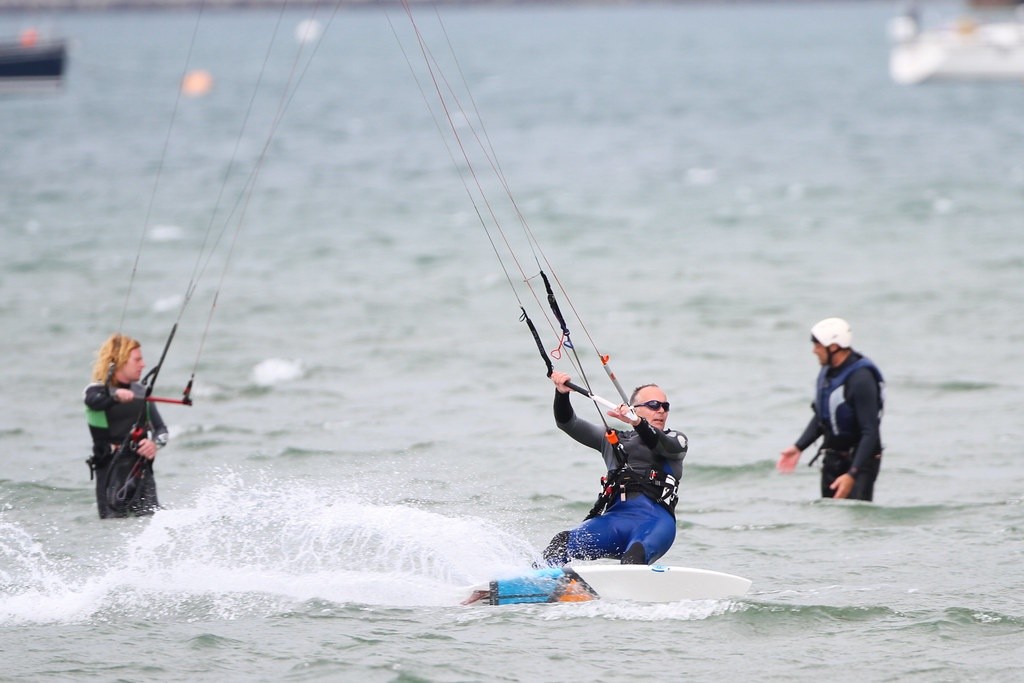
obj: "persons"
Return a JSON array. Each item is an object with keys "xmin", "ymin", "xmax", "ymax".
[
  {"xmin": 775, "ymin": 317, "xmax": 884, "ymax": 502},
  {"xmin": 540, "ymin": 369, "xmax": 688, "ymax": 568},
  {"xmin": 84, "ymin": 335, "xmax": 170, "ymax": 519}
]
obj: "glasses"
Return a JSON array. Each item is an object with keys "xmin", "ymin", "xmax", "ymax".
[{"xmin": 634, "ymin": 400, "xmax": 669, "ymax": 412}]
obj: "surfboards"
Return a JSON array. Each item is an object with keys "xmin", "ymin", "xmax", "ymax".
[{"xmin": 457, "ymin": 564, "xmax": 753, "ymax": 607}]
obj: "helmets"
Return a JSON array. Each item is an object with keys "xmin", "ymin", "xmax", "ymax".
[{"xmin": 811, "ymin": 318, "xmax": 853, "ymax": 349}]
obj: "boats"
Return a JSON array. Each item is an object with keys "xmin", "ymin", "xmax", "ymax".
[{"xmin": 887, "ymin": 21, "xmax": 1024, "ymax": 87}]
